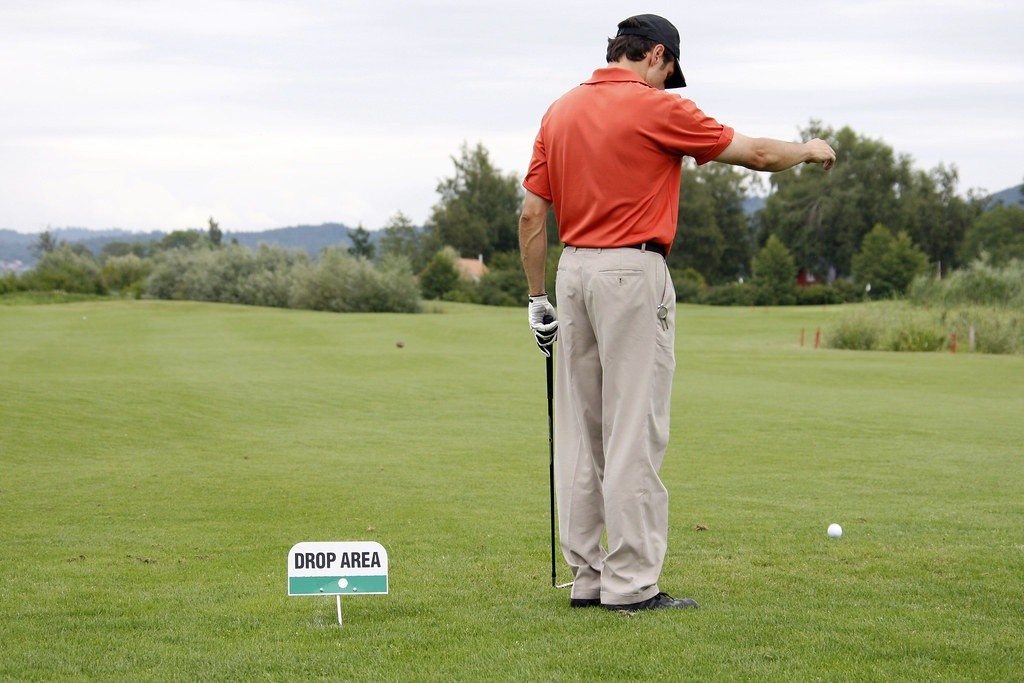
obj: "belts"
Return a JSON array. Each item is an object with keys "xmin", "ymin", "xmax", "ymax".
[{"xmin": 627, "ymin": 241, "xmax": 666, "ymax": 259}]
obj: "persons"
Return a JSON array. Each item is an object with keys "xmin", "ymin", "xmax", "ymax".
[{"xmin": 519, "ymin": 14, "xmax": 837, "ymax": 613}]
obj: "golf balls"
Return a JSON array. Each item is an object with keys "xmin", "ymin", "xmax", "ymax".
[{"xmin": 827, "ymin": 523, "xmax": 843, "ymax": 538}]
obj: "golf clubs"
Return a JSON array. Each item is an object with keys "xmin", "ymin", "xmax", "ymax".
[{"xmin": 542, "ymin": 314, "xmax": 574, "ymax": 589}]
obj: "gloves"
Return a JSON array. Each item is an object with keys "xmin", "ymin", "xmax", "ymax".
[{"xmin": 528, "ymin": 292, "xmax": 558, "ymax": 357}]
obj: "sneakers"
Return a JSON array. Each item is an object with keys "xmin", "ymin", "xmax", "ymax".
[{"xmin": 571, "ymin": 592, "xmax": 701, "ymax": 610}]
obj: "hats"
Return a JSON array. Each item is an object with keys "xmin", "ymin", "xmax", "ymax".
[{"xmin": 617, "ymin": 14, "xmax": 687, "ymax": 89}]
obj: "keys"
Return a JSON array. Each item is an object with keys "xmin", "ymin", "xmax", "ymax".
[{"xmin": 657, "ymin": 303, "xmax": 668, "ymax": 331}]
